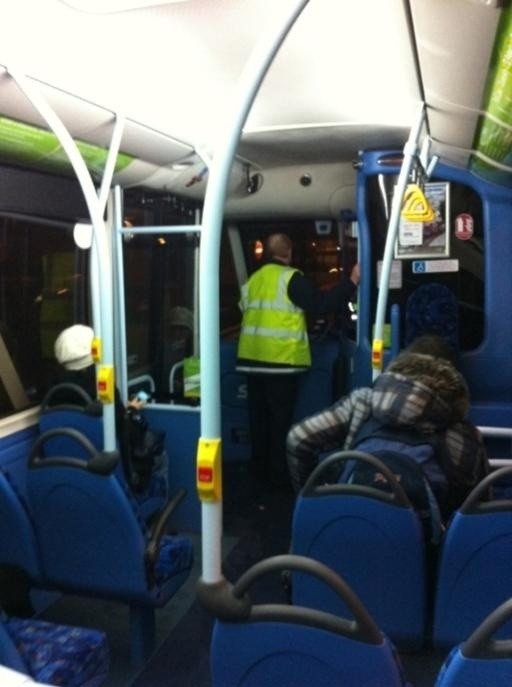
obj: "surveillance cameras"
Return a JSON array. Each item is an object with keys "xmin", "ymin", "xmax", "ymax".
[{"xmin": 301, "ymin": 174, "xmax": 311, "ymax": 186}]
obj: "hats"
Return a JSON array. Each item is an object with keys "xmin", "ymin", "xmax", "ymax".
[{"xmin": 54, "ymin": 322, "xmax": 97, "ymax": 371}]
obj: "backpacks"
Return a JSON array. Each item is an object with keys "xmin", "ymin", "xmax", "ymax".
[{"xmin": 331, "ymin": 424, "xmax": 461, "ymax": 504}]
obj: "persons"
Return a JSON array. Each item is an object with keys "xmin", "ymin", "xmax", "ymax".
[
  {"xmin": 36, "ymin": 324, "xmax": 166, "ymax": 494},
  {"xmin": 234, "ymin": 234, "xmax": 361, "ymax": 499},
  {"xmin": 285, "ymin": 332, "xmax": 496, "ymax": 504}
]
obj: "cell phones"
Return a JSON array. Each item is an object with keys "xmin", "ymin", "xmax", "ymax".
[{"xmin": 133, "ymin": 390, "xmax": 152, "ymax": 402}]
